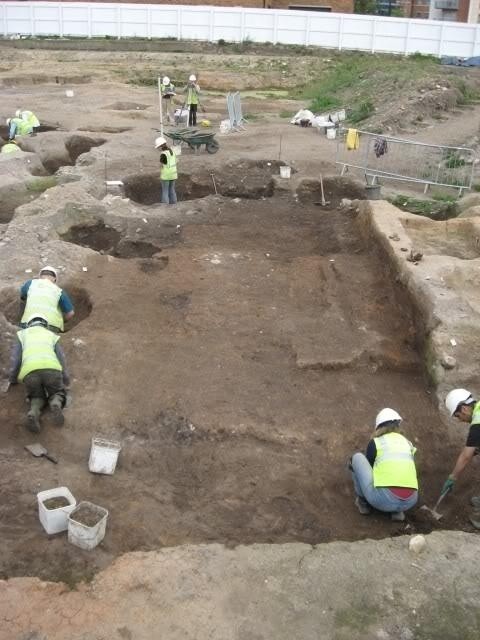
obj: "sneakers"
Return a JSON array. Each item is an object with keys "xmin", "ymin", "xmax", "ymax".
[
  {"xmin": 355, "ymin": 496, "xmax": 371, "ymax": 515},
  {"xmin": 391, "ymin": 512, "xmax": 406, "ymax": 522}
]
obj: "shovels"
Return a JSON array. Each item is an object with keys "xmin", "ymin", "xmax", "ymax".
[
  {"xmin": 420, "ymin": 488, "xmax": 450, "ymax": 520},
  {"xmin": 26, "ymin": 443, "xmax": 58, "ymax": 464}
]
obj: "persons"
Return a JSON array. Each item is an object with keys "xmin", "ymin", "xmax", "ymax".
[
  {"xmin": 154, "ymin": 137, "xmax": 177, "ymax": 203},
  {"xmin": 184, "ymin": 74, "xmax": 200, "ymax": 126},
  {"xmin": 8, "ymin": 315, "xmax": 70, "ymax": 432},
  {"xmin": 18, "ymin": 266, "xmax": 74, "ymax": 331},
  {"xmin": 2, "ymin": 139, "xmax": 21, "ymax": 152},
  {"xmin": 352, "ymin": 408, "xmax": 418, "ymax": 520},
  {"xmin": 442, "ymin": 388, "xmax": 480, "ymax": 495},
  {"xmin": 161, "ymin": 77, "xmax": 177, "ymax": 123},
  {"xmin": 15, "ymin": 109, "xmax": 40, "ymax": 131},
  {"xmin": 7, "ymin": 118, "xmax": 33, "ymax": 139}
]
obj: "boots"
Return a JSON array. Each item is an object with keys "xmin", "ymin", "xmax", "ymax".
[
  {"xmin": 49, "ymin": 395, "xmax": 64, "ymax": 425},
  {"xmin": 26, "ymin": 397, "xmax": 44, "ymax": 433}
]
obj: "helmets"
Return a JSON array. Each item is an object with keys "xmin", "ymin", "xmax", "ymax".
[
  {"xmin": 188, "ymin": 75, "xmax": 196, "ymax": 81},
  {"xmin": 375, "ymin": 408, "xmax": 402, "ymax": 430},
  {"xmin": 6, "ymin": 119, "xmax": 11, "ymax": 127},
  {"xmin": 27, "ymin": 313, "xmax": 49, "ymax": 328},
  {"xmin": 154, "ymin": 137, "xmax": 166, "ymax": 149},
  {"xmin": 163, "ymin": 77, "xmax": 170, "ymax": 85},
  {"xmin": 39, "ymin": 266, "xmax": 58, "ymax": 280},
  {"xmin": 15, "ymin": 111, "xmax": 20, "ymax": 118},
  {"xmin": 445, "ymin": 388, "xmax": 472, "ymax": 417}
]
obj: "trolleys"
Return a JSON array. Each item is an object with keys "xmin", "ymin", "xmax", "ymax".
[
  {"xmin": 226, "ymin": 91, "xmax": 249, "ymax": 132},
  {"xmin": 151, "ymin": 127, "xmax": 219, "ymax": 154}
]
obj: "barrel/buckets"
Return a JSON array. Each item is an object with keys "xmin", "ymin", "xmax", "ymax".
[{"xmin": 364, "ymin": 176, "xmax": 380, "ymax": 199}]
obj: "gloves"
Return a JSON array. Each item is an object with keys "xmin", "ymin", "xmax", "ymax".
[{"xmin": 440, "ymin": 479, "xmax": 456, "ymax": 494}]
